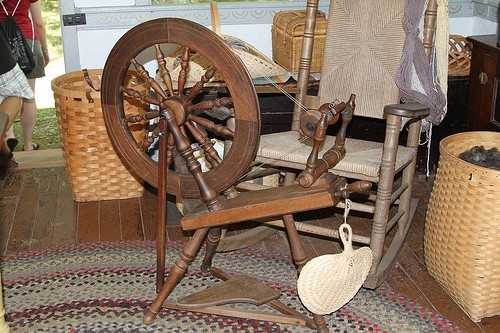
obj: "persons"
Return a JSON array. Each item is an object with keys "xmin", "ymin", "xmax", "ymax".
[{"xmin": 0, "ymin": 0, "xmax": 50, "ymax": 167}]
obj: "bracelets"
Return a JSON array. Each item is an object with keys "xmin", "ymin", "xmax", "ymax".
[{"xmin": 42, "ymin": 50, "xmax": 48, "ymax": 54}]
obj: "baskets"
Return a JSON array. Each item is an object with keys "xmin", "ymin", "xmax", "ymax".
[
  {"xmin": 423, "ymin": 130, "xmax": 500, "ymax": 323},
  {"xmin": 446, "ymin": 35, "xmax": 472, "ymax": 77},
  {"xmin": 51, "ymin": 69, "xmax": 150, "ymax": 202}
]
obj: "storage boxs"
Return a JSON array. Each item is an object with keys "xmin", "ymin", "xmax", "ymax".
[{"xmin": 271, "ymin": 8, "xmax": 330, "ymax": 75}]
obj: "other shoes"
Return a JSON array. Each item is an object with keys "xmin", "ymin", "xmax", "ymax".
[
  {"xmin": 22, "ymin": 142, "xmax": 39, "ymax": 151},
  {"xmin": 7, "ymin": 137, "xmax": 19, "ymax": 152},
  {"xmin": 0, "ymin": 112, "xmax": 10, "ymax": 140},
  {"xmin": 0, "ymin": 150, "xmax": 18, "ymax": 168}
]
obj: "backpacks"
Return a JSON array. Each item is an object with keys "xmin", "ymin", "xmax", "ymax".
[{"xmin": 0, "ymin": 0, "xmax": 35, "ymax": 75}]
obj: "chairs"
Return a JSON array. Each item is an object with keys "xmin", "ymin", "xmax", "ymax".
[{"xmin": 253, "ymin": 0, "xmax": 440, "ymax": 290}]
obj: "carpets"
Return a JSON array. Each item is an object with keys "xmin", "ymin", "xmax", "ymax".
[{"xmin": 0, "ymin": 239, "xmax": 464, "ymax": 333}]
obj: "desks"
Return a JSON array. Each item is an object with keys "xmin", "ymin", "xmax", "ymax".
[{"xmin": 466, "ymin": 35, "xmax": 500, "ymax": 133}]
